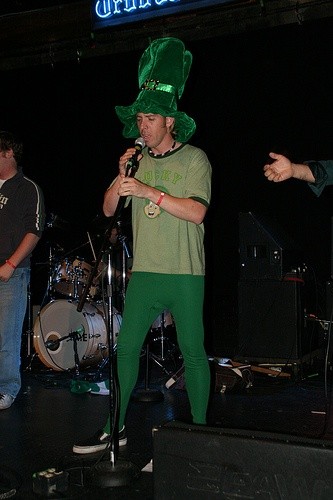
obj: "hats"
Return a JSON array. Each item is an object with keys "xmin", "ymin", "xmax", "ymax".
[{"xmin": 115, "ymin": 38, "xmax": 196, "ymax": 142}]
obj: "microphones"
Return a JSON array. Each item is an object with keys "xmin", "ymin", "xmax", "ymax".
[{"xmin": 126, "ymin": 137, "xmax": 145, "ymax": 168}]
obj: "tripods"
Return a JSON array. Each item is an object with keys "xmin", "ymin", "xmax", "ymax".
[{"xmin": 148, "ymin": 314, "xmax": 183, "ymax": 375}]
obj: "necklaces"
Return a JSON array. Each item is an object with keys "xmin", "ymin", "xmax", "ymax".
[{"xmin": 149, "ymin": 140, "xmax": 177, "ymax": 156}]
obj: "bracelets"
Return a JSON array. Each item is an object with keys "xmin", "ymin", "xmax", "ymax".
[
  {"xmin": 155, "ymin": 192, "xmax": 164, "ymax": 207},
  {"xmin": 4, "ymin": 259, "xmax": 16, "ymax": 269}
]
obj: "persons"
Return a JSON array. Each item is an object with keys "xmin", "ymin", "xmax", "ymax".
[
  {"xmin": 97, "ymin": 228, "xmax": 132, "ymax": 280},
  {"xmin": 263, "ymin": 154, "xmax": 333, "ymax": 196},
  {"xmin": 0, "ymin": 136, "xmax": 46, "ymax": 410},
  {"xmin": 72, "ymin": 39, "xmax": 211, "ymax": 456}
]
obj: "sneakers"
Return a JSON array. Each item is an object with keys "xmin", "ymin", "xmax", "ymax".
[
  {"xmin": 73, "ymin": 425, "xmax": 128, "ymax": 454},
  {"xmin": 0, "ymin": 394, "xmax": 15, "ymax": 410}
]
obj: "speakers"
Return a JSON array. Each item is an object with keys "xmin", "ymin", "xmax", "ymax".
[
  {"xmin": 152, "ymin": 422, "xmax": 333, "ymax": 500},
  {"xmin": 203, "ymin": 277, "xmax": 321, "ymax": 360}
]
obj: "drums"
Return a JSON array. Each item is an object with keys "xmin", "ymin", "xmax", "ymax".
[
  {"xmin": 31, "ymin": 299, "xmax": 122, "ymax": 372},
  {"xmin": 147, "ymin": 309, "xmax": 175, "ymax": 333},
  {"xmin": 50, "ymin": 254, "xmax": 100, "ymax": 301}
]
